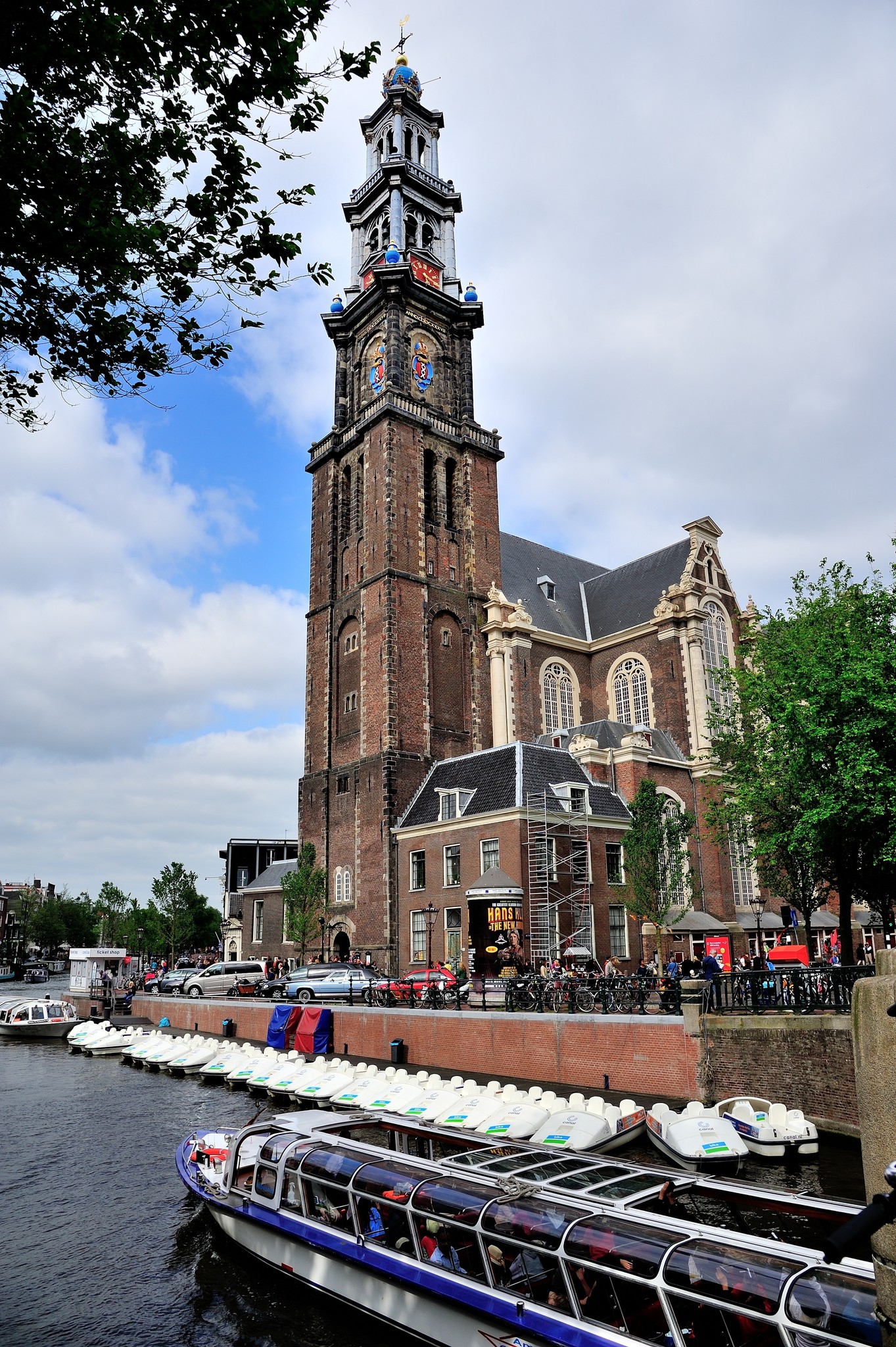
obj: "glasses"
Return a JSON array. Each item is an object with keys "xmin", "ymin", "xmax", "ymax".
[
  {"xmin": 715, "ymin": 953, "xmax": 717, "ymax": 956},
  {"xmin": 354, "ymin": 960, "xmax": 357, "ymax": 961}
]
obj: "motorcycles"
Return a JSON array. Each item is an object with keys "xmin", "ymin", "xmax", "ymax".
[{"xmin": 224, "ymin": 971, "xmax": 269, "ymax": 999}]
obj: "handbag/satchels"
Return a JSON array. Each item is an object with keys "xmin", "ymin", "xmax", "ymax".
[
  {"xmin": 668, "ymin": 971, "xmax": 672, "ymax": 976},
  {"xmin": 653, "ymin": 967, "xmax": 658, "ymax": 975},
  {"xmin": 163, "ymin": 962, "xmax": 167, "ymax": 966}
]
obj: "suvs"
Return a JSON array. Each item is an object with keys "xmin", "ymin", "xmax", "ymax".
[{"xmin": 261, "ymin": 961, "xmax": 400, "ymax": 1005}]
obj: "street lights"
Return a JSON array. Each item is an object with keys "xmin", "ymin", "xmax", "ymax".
[
  {"xmin": 318, "ymin": 917, "xmax": 325, "ymax": 963},
  {"xmin": 135, "ymin": 925, "xmax": 145, "ymax": 974},
  {"xmin": 750, "ymin": 895, "xmax": 768, "ymax": 971},
  {"xmin": 420, "ymin": 901, "xmax": 441, "ymax": 970},
  {"xmin": 219, "ymin": 919, "xmax": 232, "ymax": 962}
]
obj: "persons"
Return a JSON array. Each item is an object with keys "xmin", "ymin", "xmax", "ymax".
[
  {"xmin": 101, "ymin": 937, "xmax": 873, "ymax": 1008},
  {"xmin": 254, "ymin": 1128, "xmax": 836, "ymax": 1347},
  {"xmin": 0, "ymin": 1009, "xmax": 52, "ymax": 1023},
  {"xmin": 494, "ymin": 927, "xmax": 524, "ymax": 967}
]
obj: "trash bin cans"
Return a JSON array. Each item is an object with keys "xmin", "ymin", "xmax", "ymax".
[
  {"xmin": 222, "ymin": 1018, "xmax": 234, "ymax": 1037},
  {"xmin": 391, "ymin": 1038, "xmax": 404, "ymax": 1064}
]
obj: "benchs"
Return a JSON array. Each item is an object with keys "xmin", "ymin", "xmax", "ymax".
[{"xmin": 319, "ymin": 1171, "xmax": 846, "ymax": 1347}]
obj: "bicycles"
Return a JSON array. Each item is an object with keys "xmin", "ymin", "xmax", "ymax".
[
  {"xmin": 365, "ymin": 956, "xmax": 863, "ymax": 1016},
  {"xmin": 116, "ymin": 957, "xmax": 204, "ymax": 997}
]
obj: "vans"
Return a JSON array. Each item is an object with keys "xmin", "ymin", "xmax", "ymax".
[{"xmin": 182, "ymin": 960, "xmax": 273, "ymax": 999}]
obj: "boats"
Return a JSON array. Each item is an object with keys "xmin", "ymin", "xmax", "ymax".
[
  {"xmin": 646, "ymin": 1101, "xmax": 751, "ymax": 1175},
  {"xmin": 474, "ymin": 1086, "xmax": 569, "ymax": 1138},
  {"xmin": 433, "ymin": 1080, "xmax": 529, "ymax": 1129},
  {"xmin": 0, "ymin": 957, "xmax": 66, "ymax": 983},
  {"xmin": 173, "ymin": 1102, "xmax": 896, "ymax": 1347},
  {"xmin": 0, "ymin": 992, "xmax": 85, "ymax": 1038},
  {"xmin": 527, "ymin": 1093, "xmax": 647, "ymax": 1154},
  {"xmin": 713, "ymin": 1096, "xmax": 819, "ymax": 1158},
  {"xmin": 65, "ymin": 1020, "xmax": 465, "ymax": 1124}
]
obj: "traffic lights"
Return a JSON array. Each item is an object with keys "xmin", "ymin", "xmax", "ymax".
[
  {"xmin": 888, "ymin": 911, "xmax": 896, "ymax": 931},
  {"xmin": 780, "ymin": 905, "xmax": 793, "ymax": 926}
]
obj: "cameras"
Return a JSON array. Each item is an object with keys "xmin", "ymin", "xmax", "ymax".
[{"xmin": 664, "ymin": 1176, "xmax": 676, "ymax": 1194}]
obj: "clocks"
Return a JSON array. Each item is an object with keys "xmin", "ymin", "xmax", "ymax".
[
  {"xmin": 410, "ymin": 255, "xmax": 440, "ymax": 291},
  {"xmin": 363, "ymin": 258, "xmax": 384, "ymax": 290}
]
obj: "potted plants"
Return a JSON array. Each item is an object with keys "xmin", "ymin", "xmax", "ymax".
[
  {"xmin": 418, "ymin": 867, "xmax": 422, "ymax": 887},
  {"xmin": 453, "ymin": 874, "xmax": 458, "ymax": 883}
]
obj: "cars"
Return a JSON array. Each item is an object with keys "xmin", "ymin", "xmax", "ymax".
[
  {"xmin": 364, "ymin": 965, "xmax": 461, "ymax": 1004},
  {"xmin": 140, "ymin": 964, "xmax": 151, "ymax": 973},
  {"xmin": 143, "ymin": 968, "xmax": 205, "ymax": 996}
]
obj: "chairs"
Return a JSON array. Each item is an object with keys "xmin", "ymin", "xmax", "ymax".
[
  {"xmin": 84, "ymin": 1018, "xmax": 637, "ymax": 1120},
  {"xmin": 650, "ymin": 1100, "xmax": 717, "ymax": 1126},
  {"xmin": 731, "ymin": 1103, "xmax": 804, "ymax": 1124},
  {"xmin": 768, "ymin": 962, "xmax": 775, "ymax": 976}
]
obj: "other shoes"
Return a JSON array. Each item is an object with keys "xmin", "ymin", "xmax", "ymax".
[
  {"xmin": 123, "ymin": 1003, "xmax": 128, "ymax": 1004},
  {"xmin": 128, "ymin": 1004, "xmax": 131, "ymax": 1008},
  {"xmin": 108, "ymin": 995, "xmax": 111, "ymax": 997},
  {"xmin": 102, "ymin": 996, "xmax": 105, "ymax": 997}
]
